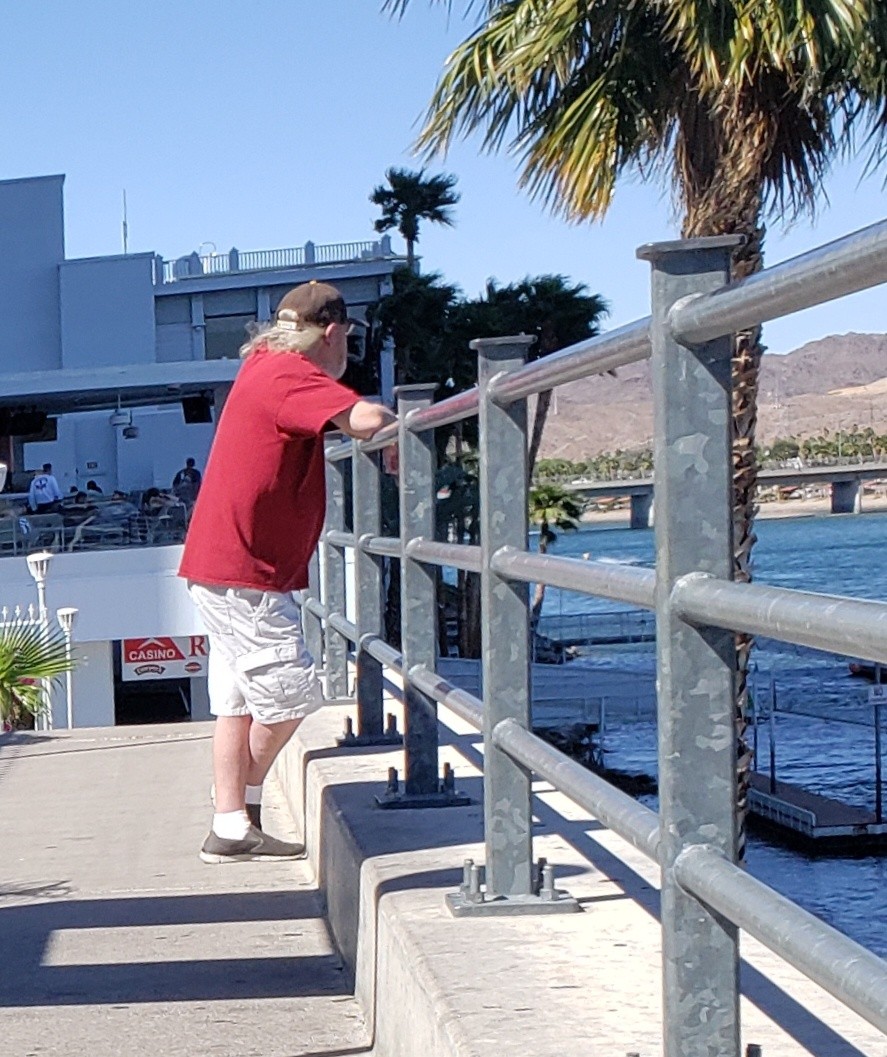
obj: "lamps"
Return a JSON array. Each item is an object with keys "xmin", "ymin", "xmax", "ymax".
[
  {"xmin": 123, "ymin": 411, "xmax": 140, "ymax": 440},
  {"xmin": 109, "ymin": 392, "xmax": 130, "ymax": 426}
]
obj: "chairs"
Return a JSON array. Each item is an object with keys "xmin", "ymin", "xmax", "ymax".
[{"xmin": 0, "ymin": 489, "xmax": 195, "ymax": 555}]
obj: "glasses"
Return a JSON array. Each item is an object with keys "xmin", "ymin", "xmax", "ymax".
[{"xmin": 319, "ymin": 322, "xmax": 354, "ymax": 336}]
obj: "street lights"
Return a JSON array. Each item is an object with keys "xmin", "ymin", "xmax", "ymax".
[
  {"xmin": 56, "ymin": 607, "xmax": 78, "ymax": 734},
  {"xmin": 27, "ymin": 549, "xmax": 52, "ymax": 732}
]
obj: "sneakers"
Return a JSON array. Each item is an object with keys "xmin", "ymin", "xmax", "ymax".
[
  {"xmin": 211, "ymin": 783, "xmax": 262, "ymax": 831},
  {"xmin": 200, "ymin": 824, "xmax": 306, "ymax": 865}
]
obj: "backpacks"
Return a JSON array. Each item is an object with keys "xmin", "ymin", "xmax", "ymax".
[{"xmin": 177, "ymin": 470, "xmax": 194, "ymax": 496}]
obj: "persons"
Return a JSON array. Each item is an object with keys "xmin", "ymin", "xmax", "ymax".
[
  {"xmin": 143, "ymin": 488, "xmax": 168, "ymax": 517},
  {"xmin": 172, "ymin": 458, "xmax": 202, "ymax": 529},
  {"xmin": 178, "ymin": 278, "xmax": 399, "ymax": 873},
  {"xmin": 69, "ymin": 481, "xmax": 105, "ymax": 504},
  {"xmin": 29, "ymin": 463, "xmax": 64, "ymax": 512}
]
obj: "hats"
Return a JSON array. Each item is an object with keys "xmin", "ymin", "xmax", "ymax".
[{"xmin": 275, "ymin": 281, "xmax": 370, "ymax": 331}]
